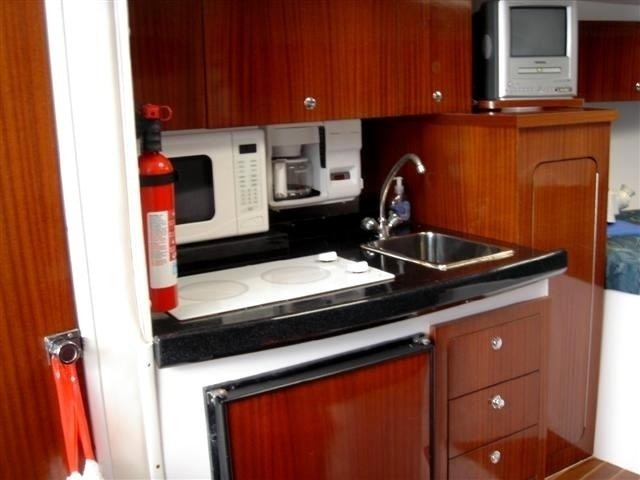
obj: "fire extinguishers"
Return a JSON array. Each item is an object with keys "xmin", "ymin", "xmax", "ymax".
[{"xmin": 138, "ymin": 103, "xmax": 179, "ymax": 313}]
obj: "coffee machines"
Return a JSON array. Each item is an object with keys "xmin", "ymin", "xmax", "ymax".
[{"xmin": 266, "ymin": 116, "xmax": 365, "ymax": 211}]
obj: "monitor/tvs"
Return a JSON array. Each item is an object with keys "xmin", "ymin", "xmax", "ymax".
[{"xmin": 473, "ymin": 0, "xmax": 578, "ymax": 98}]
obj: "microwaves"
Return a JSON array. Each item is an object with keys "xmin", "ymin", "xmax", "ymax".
[{"xmin": 138, "ymin": 124, "xmax": 268, "ymax": 242}]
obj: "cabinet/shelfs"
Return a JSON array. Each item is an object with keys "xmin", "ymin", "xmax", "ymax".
[
  {"xmin": 573, "ymin": 18, "xmax": 638, "ymax": 105},
  {"xmin": 430, "ymin": 289, "xmax": 559, "ymax": 480},
  {"xmin": 200, "ymin": 1, "xmax": 473, "ymax": 132},
  {"xmin": 369, "ymin": 106, "xmax": 622, "ymax": 480},
  {"xmin": 196, "ymin": 329, "xmax": 437, "ymax": 480},
  {"xmin": 127, "ymin": 1, "xmax": 208, "ymax": 138}
]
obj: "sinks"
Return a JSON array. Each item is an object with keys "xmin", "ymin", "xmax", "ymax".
[{"xmin": 360, "ymin": 230, "xmax": 515, "ymax": 272}]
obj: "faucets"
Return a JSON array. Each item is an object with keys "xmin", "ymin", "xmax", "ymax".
[{"xmin": 361, "ymin": 151, "xmax": 427, "ymax": 239}]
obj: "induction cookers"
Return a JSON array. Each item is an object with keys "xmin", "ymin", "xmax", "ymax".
[{"xmin": 166, "ymin": 246, "xmax": 397, "ymax": 323}]
